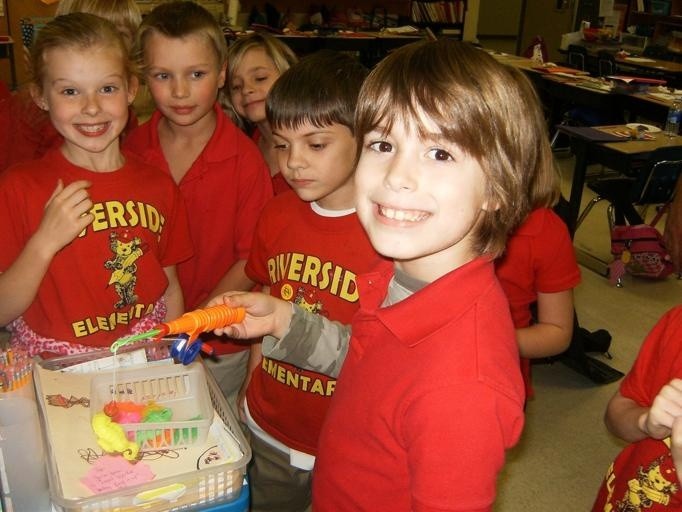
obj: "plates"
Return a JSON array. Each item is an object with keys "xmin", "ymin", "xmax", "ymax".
[{"xmin": 626, "ymin": 123, "xmax": 661, "ymax": 134}]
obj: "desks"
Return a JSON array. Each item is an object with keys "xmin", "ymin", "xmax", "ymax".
[{"xmin": 219, "ymin": 15, "xmax": 682, "ymax": 285}]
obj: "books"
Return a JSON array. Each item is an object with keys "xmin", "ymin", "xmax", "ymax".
[{"xmin": 377, "ymin": 0, "xmax": 468, "ymax": 41}]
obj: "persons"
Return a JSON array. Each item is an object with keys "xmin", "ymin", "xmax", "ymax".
[
  {"xmin": 310, "ymin": 39, "xmax": 558, "ymax": 510},
  {"xmin": 118, "ymin": 0, "xmax": 271, "ymax": 432},
  {"xmin": 588, "ymin": 294, "xmax": 682, "ymax": 511},
  {"xmin": 1, "ymin": 10, "xmax": 195, "ymax": 387},
  {"xmin": 224, "ymin": 32, "xmax": 298, "ymax": 181},
  {"xmin": 237, "ymin": 50, "xmax": 381, "ymax": 511},
  {"xmin": 493, "ymin": 208, "xmax": 584, "ymax": 411},
  {"xmin": 0, "ymin": 1, "xmax": 144, "ymax": 154}
]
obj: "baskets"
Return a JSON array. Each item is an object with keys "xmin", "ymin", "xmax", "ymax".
[
  {"xmin": 89, "ymin": 361, "xmax": 214, "ymax": 453},
  {"xmin": 31, "ymin": 340, "xmax": 253, "ymax": 512}
]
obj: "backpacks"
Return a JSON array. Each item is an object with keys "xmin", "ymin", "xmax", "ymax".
[{"xmin": 608, "ymin": 224, "xmax": 674, "ymax": 282}]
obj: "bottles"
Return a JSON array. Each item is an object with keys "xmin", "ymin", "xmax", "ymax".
[
  {"xmin": 662, "ymin": 98, "xmax": 682, "ymax": 139},
  {"xmin": 370, "ymin": 7, "xmax": 385, "ymax": 33}
]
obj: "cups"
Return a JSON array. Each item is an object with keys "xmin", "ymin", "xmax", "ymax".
[{"xmin": 386, "ymin": 12, "xmax": 400, "ymax": 30}]
